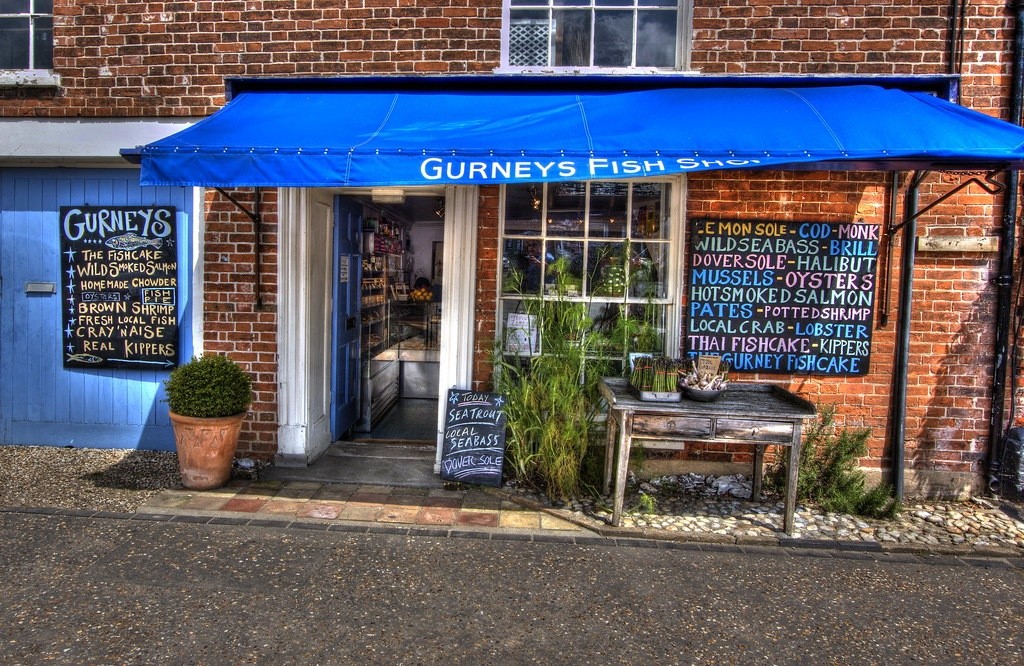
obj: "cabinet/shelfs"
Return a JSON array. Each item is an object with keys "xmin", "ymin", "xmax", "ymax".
[{"xmin": 361, "ymin": 250, "xmax": 410, "ymax": 353}]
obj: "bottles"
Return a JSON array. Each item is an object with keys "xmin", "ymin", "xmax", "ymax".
[{"xmin": 362, "ymin": 210, "xmax": 415, "ymax": 271}]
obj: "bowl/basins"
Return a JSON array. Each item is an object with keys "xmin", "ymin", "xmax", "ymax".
[{"xmin": 680, "ymin": 382, "xmax": 727, "ymax": 402}]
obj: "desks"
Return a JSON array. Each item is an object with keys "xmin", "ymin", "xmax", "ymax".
[{"xmin": 598, "ymin": 377, "xmax": 818, "ymax": 536}]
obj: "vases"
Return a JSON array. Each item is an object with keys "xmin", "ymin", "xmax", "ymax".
[{"xmin": 565, "ymin": 341, "xmax": 580, "ymax": 349}]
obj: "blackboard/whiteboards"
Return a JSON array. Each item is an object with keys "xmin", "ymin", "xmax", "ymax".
[
  {"xmin": 440, "ymin": 388, "xmax": 508, "ymax": 488},
  {"xmin": 59, "ymin": 205, "xmax": 179, "ymax": 372},
  {"xmin": 685, "ymin": 215, "xmax": 880, "ymax": 377}
]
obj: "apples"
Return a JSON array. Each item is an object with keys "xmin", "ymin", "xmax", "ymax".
[{"xmin": 410, "ymin": 287, "xmax": 433, "ymax": 301}]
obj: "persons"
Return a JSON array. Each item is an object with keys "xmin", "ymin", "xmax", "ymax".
[{"xmin": 523, "ymin": 238, "xmax": 555, "ymax": 293}]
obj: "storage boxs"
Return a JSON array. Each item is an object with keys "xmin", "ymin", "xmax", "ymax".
[{"xmin": 399, "ymin": 294, "xmax": 409, "ymax": 301}]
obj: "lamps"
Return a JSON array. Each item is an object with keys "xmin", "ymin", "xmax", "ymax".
[
  {"xmin": 529, "ymin": 184, "xmax": 540, "ymax": 209},
  {"xmin": 372, "ymin": 189, "xmax": 405, "ymax": 204},
  {"xmin": 436, "ymin": 199, "xmax": 445, "ymax": 218}
]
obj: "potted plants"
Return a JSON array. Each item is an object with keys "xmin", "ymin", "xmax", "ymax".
[{"xmin": 160, "ymin": 353, "xmax": 254, "ymax": 490}]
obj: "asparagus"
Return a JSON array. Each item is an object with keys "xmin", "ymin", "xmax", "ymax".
[
  {"xmin": 631, "ymin": 356, "xmax": 681, "ymax": 392},
  {"xmin": 719, "ymin": 361, "xmax": 731, "ymax": 379}
]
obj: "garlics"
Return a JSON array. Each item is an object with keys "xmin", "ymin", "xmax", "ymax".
[{"xmin": 680, "ymin": 372, "xmax": 727, "ymax": 391}]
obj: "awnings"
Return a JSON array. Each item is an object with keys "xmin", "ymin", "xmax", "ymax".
[{"xmin": 118, "ymin": 86, "xmax": 1024, "ymax": 241}]
